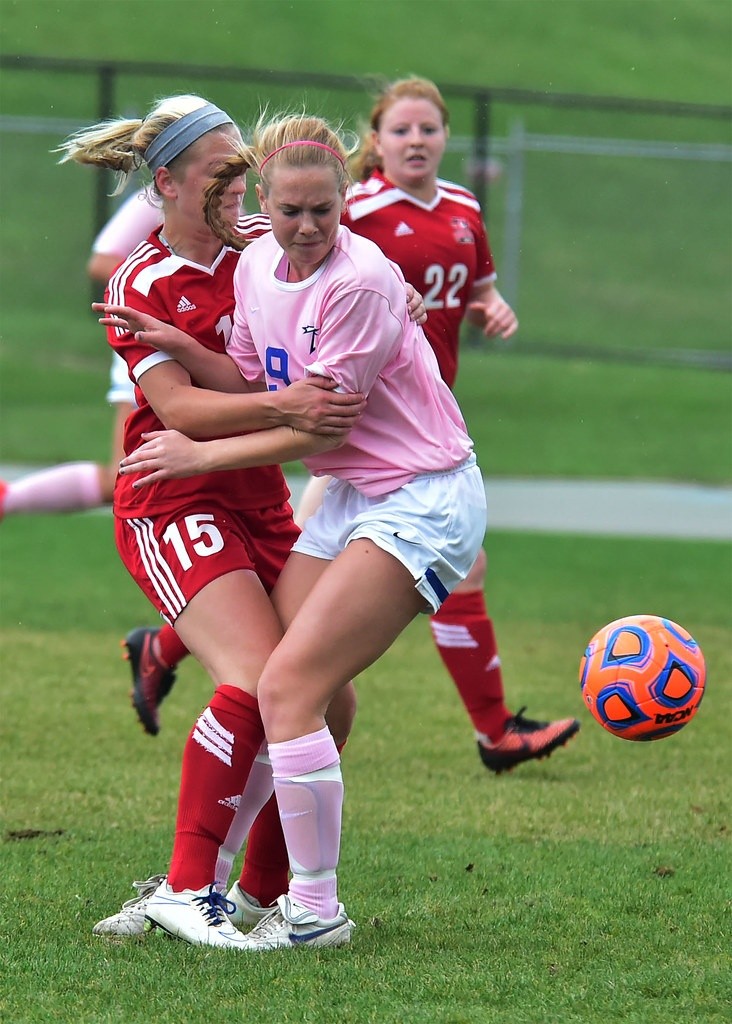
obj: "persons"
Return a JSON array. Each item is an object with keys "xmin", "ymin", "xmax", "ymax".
[
  {"xmin": 91, "ymin": 117, "xmax": 488, "ymax": 950},
  {"xmin": 123, "ymin": 78, "xmax": 579, "ymax": 772},
  {"xmin": 51, "ymin": 95, "xmax": 426, "ymax": 950},
  {"xmin": 0, "ymin": 181, "xmax": 247, "ymax": 516}
]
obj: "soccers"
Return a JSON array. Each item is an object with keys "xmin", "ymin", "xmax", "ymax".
[{"xmin": 578, "ymin": 612, "xmax": 708, "ymax": 742}]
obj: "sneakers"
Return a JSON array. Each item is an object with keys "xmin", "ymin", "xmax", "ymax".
[
  {"xmin": 478, "ymin": 705, "xmax": 580, "ymax": 777},
  {"xmin": 144, "ymin": 878, "xmax": 256, "ymax": 951},
  {"xmin": 120, "ymin": 626, "xmax": 175, "ymax": 735},
  {"xmin": 244, "ymin": 893, "xmax": 355, "ymax": 953},
  {"xmin": 92, "ymin": 873, "xmax": 168, "ymax": 937},
  {"xmin": 223, "ymin": 880, "xmax": 277, "ymax": 925}
]
